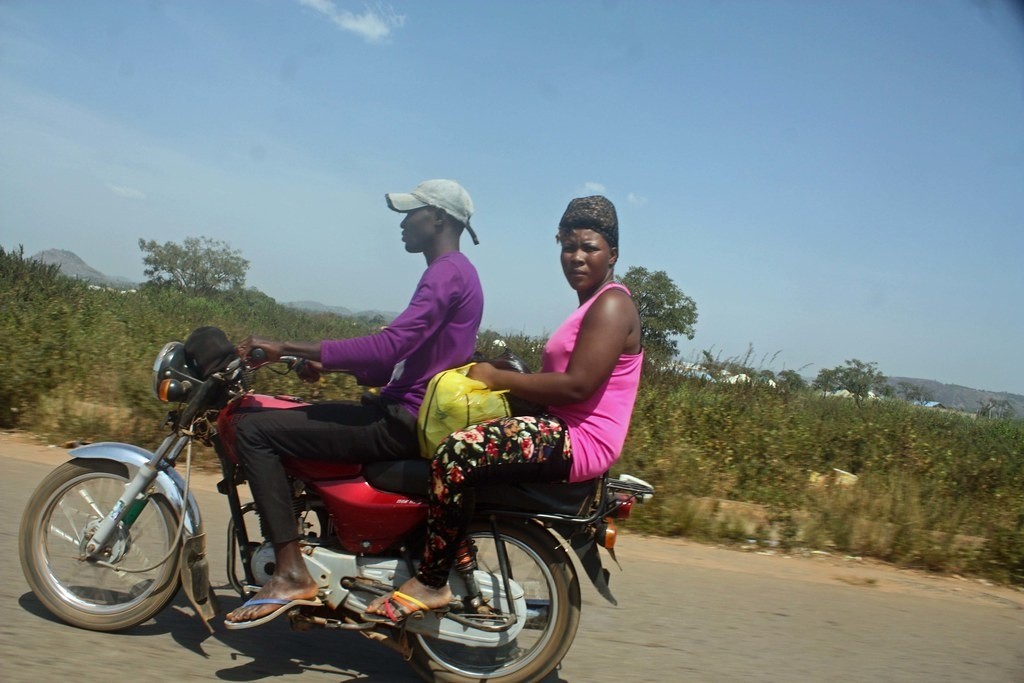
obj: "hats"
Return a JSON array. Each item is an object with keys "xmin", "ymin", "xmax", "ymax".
[
  {"xmin": 556, "ymin": 196, "xmax": 619, "ymax": 247},
  {"xmin": 382, "ymin": 177, "xmax": 482, "ymax": 246}
]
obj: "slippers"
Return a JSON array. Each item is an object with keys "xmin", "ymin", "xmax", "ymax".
[
  {"xmin": 360, "ymin": 590, "xmax": 451, "ymax": 624},
  {"xmin": 223, "ymin": 590, "xmax": 323, "ymax": 628}
]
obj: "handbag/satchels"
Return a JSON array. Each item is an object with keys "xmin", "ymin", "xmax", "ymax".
[
  {"xmin": 185, "ymin": 325, "xmax": 239, "ymax": 377},
  {"xmin": 418, "ymin": 363, "xmax": 511, "ymax": 459}
]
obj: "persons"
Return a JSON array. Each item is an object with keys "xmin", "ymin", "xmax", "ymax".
[
  {"xmin": 225, "ymin": 180, "xmax": 484, "ymax": 628},
  {"xmin": 362, "ymin": 196, "xmax": 644, "ymax": 620}
]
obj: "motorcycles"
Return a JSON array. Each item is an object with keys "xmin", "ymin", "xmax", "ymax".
[{"xmin": 17, "ymin": 324, "xmax": 660, "ymax": 682}]
